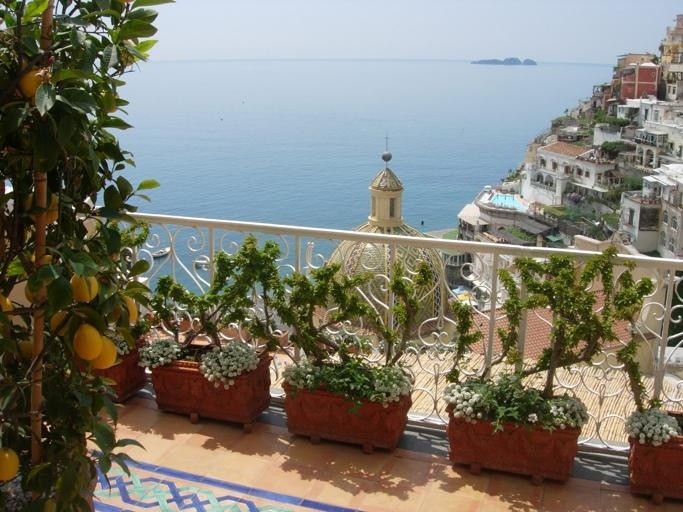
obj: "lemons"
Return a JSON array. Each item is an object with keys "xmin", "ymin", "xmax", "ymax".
[{"xmin": 0, "ymin": 64, "xmax": 137, "ymax": 512}]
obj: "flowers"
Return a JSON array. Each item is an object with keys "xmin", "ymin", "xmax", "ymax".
[
  {"xmin": 269, "ymin": 259, "xmax": 439, "ymax": 419},
  {"xmin": 613, "ymin": 260, "xmax": 683, "ymax": 446},
  {"xmin": 137, "ymin": 234, "xmax": 279, "ymax": 390},
  {"xmin": 75, "ymin": 215, "xmax": 150, "ymax": 355},
  {"xmin": 441, "ymin": 245, "xmax": 618, "ymax": 431}
]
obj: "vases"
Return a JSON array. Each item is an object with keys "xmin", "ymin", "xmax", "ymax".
[
  {"xmin": 64, "ymin": 333, "xmax": 147, "ymax": 402},
  {"xmin": 281, "ymin": 381, "xmax": 414, "ymax": 453},
  {"xmin": 0, "ymin": 441, "xmax": 99, "ymax": 512},
  {"xmin": 149, "ymin": 343, "xmax": 274, "ymax": 432},
  {"xmin": 445, "ymin": 395, "xmax": 582, "ymax": 486},
  {"xmin": 628, "ymin": 411, "xmax": 683, "ymax": 504}
]
